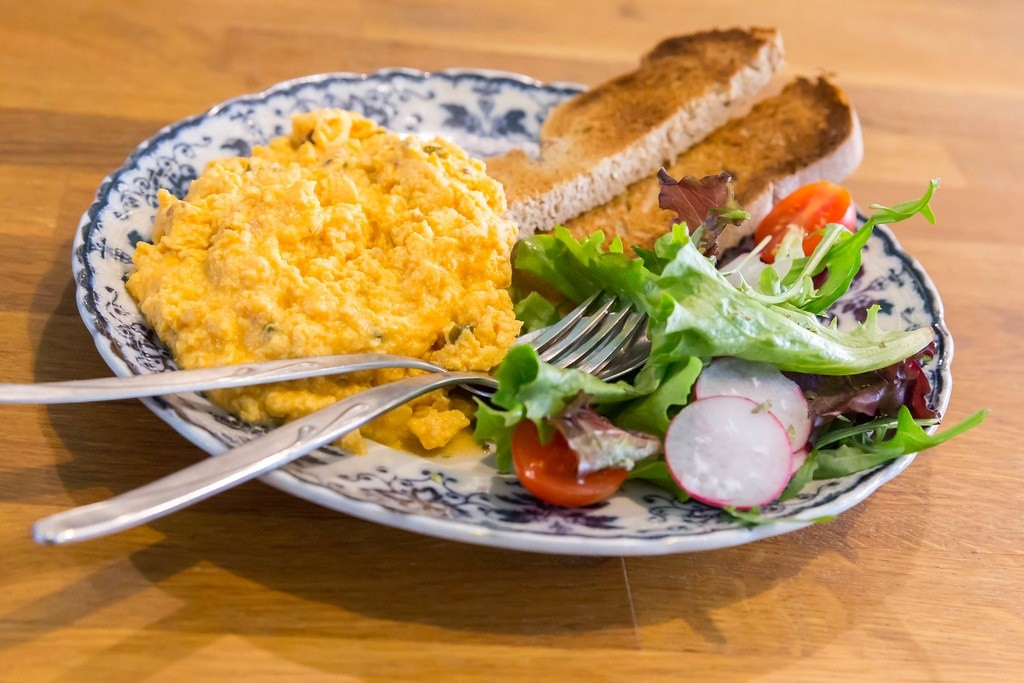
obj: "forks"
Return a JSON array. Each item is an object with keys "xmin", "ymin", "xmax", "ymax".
[{"xmin": 34, "ymin": 289, "xmax": 648, "ymax": 547}]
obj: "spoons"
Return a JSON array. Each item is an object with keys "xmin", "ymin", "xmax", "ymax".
[{"xmin": 0, "ymin": 312, "xmax": 653, "ymax": 405}]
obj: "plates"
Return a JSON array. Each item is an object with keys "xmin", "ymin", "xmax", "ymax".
[{"xmin": 72, "ymin": 67, "xmax": 955, "ymax": 557}]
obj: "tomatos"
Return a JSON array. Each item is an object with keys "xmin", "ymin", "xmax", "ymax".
[
  {"xmin": 512, "ymin": 418, "xmax": 627, "ymax": 507},
  {"xmin": 756, "ymin": 182, "xmax": 857, "ymax": 262}
]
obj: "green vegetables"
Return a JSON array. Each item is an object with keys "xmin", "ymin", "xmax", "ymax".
[{"xmin": 476, "ymin": 180, "xmax": 991, "ymax": 526}]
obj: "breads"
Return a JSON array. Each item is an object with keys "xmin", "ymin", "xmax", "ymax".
[
  {"xmin": 488, "ymin": 28, "xmax": 782, "ymax": 236},
  {"xmin": 538, "ymin": 73, "xmax": 862, "ymax": 263}
]
